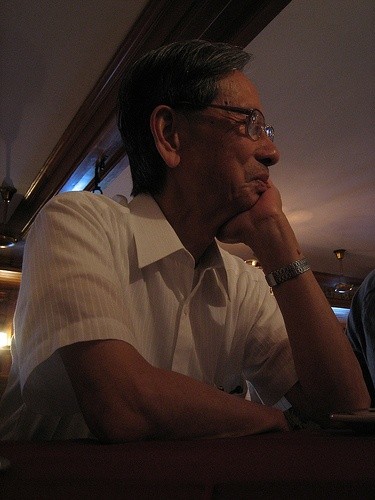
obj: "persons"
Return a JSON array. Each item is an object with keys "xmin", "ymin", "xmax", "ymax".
[
  {"xmin": 345, "ymin": 268, "xmax": 375, "ymax": 408},
  {"xmin": 0, "ymin": 40, "xmax": 371, "ymax": 442}
]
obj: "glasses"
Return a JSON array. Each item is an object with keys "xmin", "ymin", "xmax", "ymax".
[{"xmin": 168, "ymin": 102, "xmax": 275, "ymax": 143}]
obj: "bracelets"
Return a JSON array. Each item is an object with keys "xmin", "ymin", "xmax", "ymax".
[
  {"xmin": 284, "ymin": 404, "xmax": 308, "ymax": 431},
  {"xmin": 265, "ymin": 256, "xmax": 312, "ymax": 287}
]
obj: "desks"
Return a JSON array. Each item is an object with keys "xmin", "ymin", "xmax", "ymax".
[{"xmin": 0, "ymin": 427, "xmax": 375, "ymax": 500}]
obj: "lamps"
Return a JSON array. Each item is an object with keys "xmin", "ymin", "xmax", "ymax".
[
  {"xmin": 330, "ymin": 249, "xmax": 354, "ymax": 294},
  {"xmin": 0, "ymin": 186, "xmax": 17, "ymax": 249}
]
